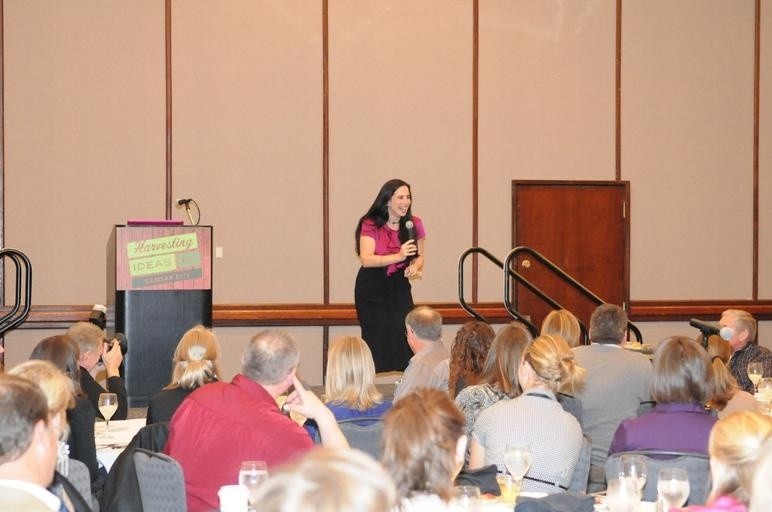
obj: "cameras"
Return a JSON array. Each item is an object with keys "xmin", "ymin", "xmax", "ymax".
[{"xmin": 88, "ymin": 305, "xmax": 128, "ymax": 363}]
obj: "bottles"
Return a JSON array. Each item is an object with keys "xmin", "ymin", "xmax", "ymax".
[{"xmin": 219, "ymin": 485, "xmax": 249, "ymax": 511}]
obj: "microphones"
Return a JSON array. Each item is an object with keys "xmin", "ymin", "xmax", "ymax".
[
  {"xmin": 405, "ymin": 221, "xmax": 414, "ymax": 253},
  {"xmin": 175, "ymin": 198, "xmax": 192, "ymax": 209},
  {"xmin": 690, "ymin": 318, "xmax": 734, "ymax": 341}
]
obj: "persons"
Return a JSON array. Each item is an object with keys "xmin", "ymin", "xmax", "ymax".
[
  {"xmin": 354, "ymin": 180, "xmax": 425, "ymax": 374},
  {"xmin": 0, "ymin": 303, "xmax": 772, "ymax": 512}
]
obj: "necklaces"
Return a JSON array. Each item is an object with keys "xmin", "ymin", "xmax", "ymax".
[{"xmin": 388, "ymin": 219, "xmax": 401, "ymax": 225}]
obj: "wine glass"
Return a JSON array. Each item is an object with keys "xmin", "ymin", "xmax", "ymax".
[
  {"xmin": 746, "ymin": 361, "xmax": 763, "ymax": 397},
  {"xmin": 99, "ymin": 391, "xmax": 119, "ymax": 442}
]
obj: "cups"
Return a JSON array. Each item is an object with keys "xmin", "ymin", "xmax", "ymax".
[
  {"xmin": 237, "ymin": 461, "xmax": 268, "ymax": 502},
  {"xmin": 655, "ymin": 467, "xmax": 692, "ymax": 510},
  {"xmin": 448, "ymin": 484, "xmax": 481, "ymax": 506},
  {"xmin": 605, "ymin": 453, "xmax": 647, "ymax": 511},
  {"xmin": 756, "ymin": 378, "xmax": 772, "ymax": 407},
  {"xmin": 503, "ymin": 443, "xmax": 533, "ymax": 494}
]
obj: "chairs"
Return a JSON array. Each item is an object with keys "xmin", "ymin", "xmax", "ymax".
[
  {"xmin": 602, "ymin": 450, "xmax": 710, "ymax": 506},
  {"xmin": 638, "ymin": 400, "xmax": 657, "ymax": 415},
  {"xmin": 313, "ymin": 418, "xmax": 397, "ymax": 458},
  {"xmin": 64, "ymin": 458, "xmax": 98, "ymax": 510},
  {"xmin": 566, "ymin": 434, "xmax": 592, "ymax": 495},
  {"xmin": 133, "ymin": 447, "xmax": 187, "ymax": 510}
]
